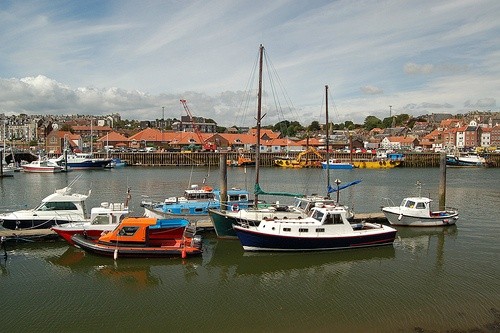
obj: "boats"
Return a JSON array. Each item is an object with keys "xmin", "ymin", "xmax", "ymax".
[
  {"xmin": 380, "ymin": 152, "xmax": 459, "ymax": 226},
  {"xmin": 319, "ymin": 159, "xmax": 354, "ymax": 169},
  {"xmin": 61, "ymin": 120, "xmax": 113, "ymax": 170},
  {"xmin": 446, "ymin": 154, "xmax": 485, "ymax": 166},
  {"xmin": 139, "ymin": 153, "xmax": 267, "ymax": 232},
  {"xmin": 50, "ymin": 186, "xmax": 133, "ymax": 249},
  {"xmin": 138, "ymin": 43, "xmax": 398, "ymax": 252},
  {"xmin": 19, "ymin": 151, "xmax": 64, "ymax": 173},
  {"xmin": 0, "ymin": 174, "xmax": 93, "ymax": 244},
  {"xmin": 70, "ymin": 213, "xmax": 203, "ymax": 259}
]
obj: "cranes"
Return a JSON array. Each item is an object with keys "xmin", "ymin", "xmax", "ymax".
[{"xmin": 179, "ymin": 99, "xmax": 218, "ymax": 150}]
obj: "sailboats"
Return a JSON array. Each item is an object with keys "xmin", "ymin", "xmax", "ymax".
[{"xmin": 2, "ymin": 123, "xmax": 15, "ymax": 177}]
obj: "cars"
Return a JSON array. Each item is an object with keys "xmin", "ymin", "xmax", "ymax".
[{"xmin": 114, "ymin": 147, "xmax": 160, "ymax": 154}]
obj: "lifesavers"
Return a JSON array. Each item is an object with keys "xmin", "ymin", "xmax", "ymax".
[{"xmin": 230, "ymin": 203, "xmax": 239, "ymax": 212}]
